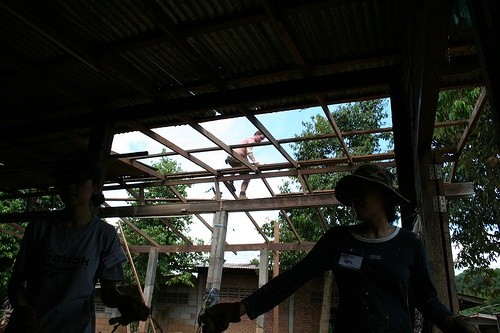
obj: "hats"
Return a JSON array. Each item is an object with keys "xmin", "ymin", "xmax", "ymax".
[{"xmin": 335, "ymin": 163, "xmax": 411, "ymax": 208}]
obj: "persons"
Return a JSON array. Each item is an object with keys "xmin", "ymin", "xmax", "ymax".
[
  {"xmin": 198, "ymin": 163, "xmax": 476, "ymax": 333},
  {"xmin": 224, "ymin": 131, "xmax": 270, "ymax": 199},
  {"xmin": 4, "ymin": 156, "xmax": 151, "ymax": 332}
]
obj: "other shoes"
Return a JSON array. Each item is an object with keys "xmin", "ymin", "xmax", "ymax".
[{"xmin": 239, "ymin": 193, "xmax": 248, "ymax": 199}]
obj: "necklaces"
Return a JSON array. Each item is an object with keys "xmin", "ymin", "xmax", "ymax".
[{"xmin": 362, "ymin": 223, "xmax": 391, "ymax": 238}]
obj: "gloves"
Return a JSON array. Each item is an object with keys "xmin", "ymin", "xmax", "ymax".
[
  {"xmin": 5, "ymin": 306, "xmax": 37, "ymax": 333},
  {"xmin": 118, "ymin": 295, "xmax": 149, "ymax": 325},
  {"xmin": 438, "ymin": 315, "xmax": 480, "ymax": 333},
  {"xmin": 200, "ymin": 302, "xmax": 240, "ymax": 333}
]
